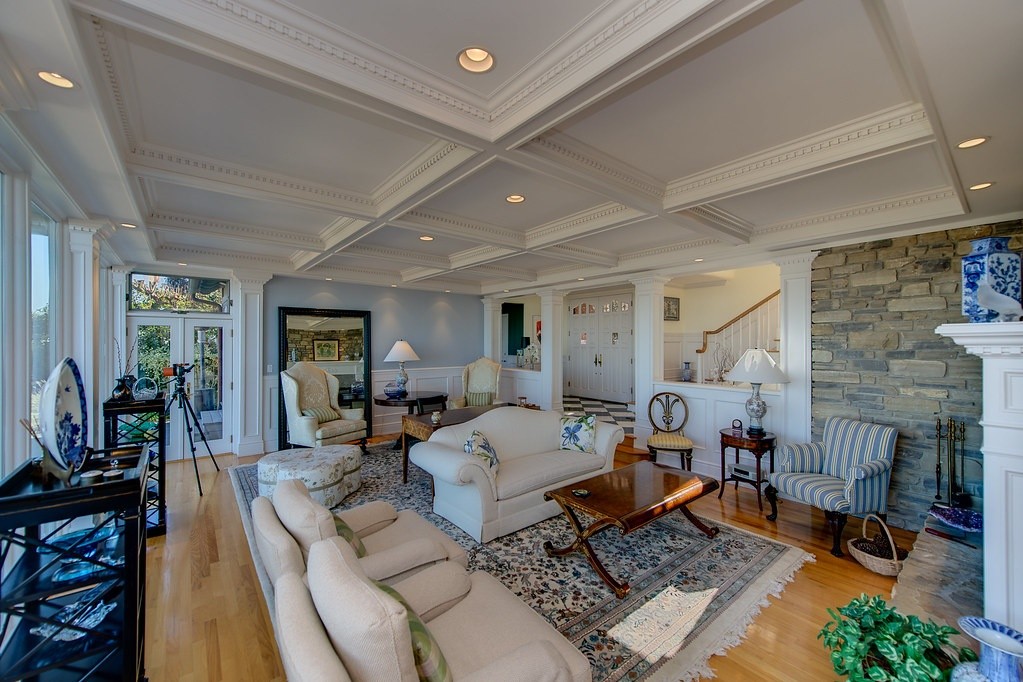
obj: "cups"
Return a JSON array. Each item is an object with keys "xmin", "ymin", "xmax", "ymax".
[
  {"xmin": 103, "ymin": 470, "xmax": 124, "ymax": 481},
  {"xmin": 80, "ymin": 470, "xmax": 103, "ymax": 485}
]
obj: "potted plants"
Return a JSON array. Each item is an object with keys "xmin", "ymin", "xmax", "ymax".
[{"xmin": 816, "ymin": 592, "xmax": 979, "ymax": 682}]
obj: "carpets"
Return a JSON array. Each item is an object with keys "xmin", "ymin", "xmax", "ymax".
[{"xmin": 227, "ymin": 441, "xmax": 813, "ymax": 682}]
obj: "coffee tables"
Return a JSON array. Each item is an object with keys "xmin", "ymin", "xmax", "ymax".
[{"xmin": 538, "ymin": 461, "xmax": 719, "ymax": 600}]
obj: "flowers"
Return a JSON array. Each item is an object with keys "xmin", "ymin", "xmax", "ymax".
[{"xmin": 523, "ymin": 343, "xmax": 539, "ymax": 363}]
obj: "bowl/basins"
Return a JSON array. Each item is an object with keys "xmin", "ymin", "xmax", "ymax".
[{"xmin": 39, "ymin": 357, "xmax": 88, "ymax": 472}]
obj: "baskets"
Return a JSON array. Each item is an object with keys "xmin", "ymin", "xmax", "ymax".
[{"xmin": 847, "ymin": 515, "xmax": 903, "ymax": 576}]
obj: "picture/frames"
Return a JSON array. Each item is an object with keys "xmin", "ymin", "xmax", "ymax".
[
  {"xmin": 314, "ymin": 337, "xmax": 340, "ymax": 362},
  {"xmin": 663, "ymin": 297, "xmax": 680, "ymax": 321}
]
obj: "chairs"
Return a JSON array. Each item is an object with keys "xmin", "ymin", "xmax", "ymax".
[
  {"xmin": 765, "ymin": 417, "xmax": 900, "ymax": 557},
  {"xmin": 278, "ymin": 362, "xmax": 369, "ymax": 447},
  {"xmin": 647, "ymin": 390, "xmax": 693, "ymax": 471},
  {"xmin": 449, "ymin": 358, "xmax": 504, "ymax": 410}
]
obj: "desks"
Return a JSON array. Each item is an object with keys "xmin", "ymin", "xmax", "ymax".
[
  {"xmin": 717, "ymin": 428, "xmax": 778, "ymax": 512},
  {"xmin": 401, "ymin": 402, "xmax": 510, "ymax": 504},
  {"xmin": 371, "ymin": 390, "xmax": 448, "ymax": 452},
  {"xmin": 341, "ymin": 393, "xmax": 365, "ymax": 409}
]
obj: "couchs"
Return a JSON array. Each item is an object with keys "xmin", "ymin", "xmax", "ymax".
[
  {"xmin": 271, "ymin": 565, "xmax": 594, "ymax": 682},
  {"xmin": 411, "ymin": 407, "xmax": 624, "ymax": 546},
  {"xmin": 244, "ymin": 496, "xmax": 469, "ymax": 584}
]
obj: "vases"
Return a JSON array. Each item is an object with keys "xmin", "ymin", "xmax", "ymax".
[
  {"xmin": 530, "ymin": 361, "xmax": 534, "ymax": 371},
  {"xmin": 949, "ymin": 615, "xmax": 1023, "ymax": 682},
  {"xmin": 112, "ymin": 377, "xmax": 134, "ymax": 402},
  {"xmin": 683, "ymin": 361, "xmax": 692, "ymax": 381}
]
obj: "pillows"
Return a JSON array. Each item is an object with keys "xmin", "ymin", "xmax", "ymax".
[
  {"xmin": 559, "ymin": 413, "xmax": 597, "ymax": 452},
  {"xmin": 367, "ymin": 574, "xmax": 454, "ymax": 682},
  {"xmin": 301, "ymin": 406, "xmax": 341, "ymax": 423},
  {"xmin": 463, "ymin": 430, "xmax": 501, "ymax": 473},
  {"xmin": 331, "ymin": 513, "xmax": 369, "ymax": 558}
]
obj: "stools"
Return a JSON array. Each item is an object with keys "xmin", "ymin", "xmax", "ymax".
[{"xmin": 255, "ymin": 443, "xmax": 365, "ymax": 509}]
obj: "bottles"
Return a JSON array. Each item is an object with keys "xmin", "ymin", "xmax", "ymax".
[
  {"xmin": 682, "ymin": 361, "xmax": 692, "ymax": 382},
  {"xmin": 430, "ymin": 411, "xmax": 441, "ymax": 424},
  {"xmin": 288, "ymin": 347, "xmax": 300, "ymax": 362},
  {"xmin": 502, "ymin": 352, "xmax": 508, "ymax": 367},
  {"xmin": 517, "ymin": 354, "xmax": 524, "ymax": 366}
]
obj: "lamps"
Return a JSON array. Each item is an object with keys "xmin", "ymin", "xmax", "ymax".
[
  {"xmin": 724, "ymin": 347, "xmax": 791, "ymax": 435},
  {"xmin": 383, "ymin": 339, "xmax": 420, "ymax": 393}
]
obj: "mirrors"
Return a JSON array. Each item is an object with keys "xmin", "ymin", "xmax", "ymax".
[{"xmin": 277, "ymin": 307, "xmax": 371, "ymax": 451}]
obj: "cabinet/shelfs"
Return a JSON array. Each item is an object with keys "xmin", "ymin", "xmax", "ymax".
[
  {"xmin": 933, "ymin": 323, "xmax": 1023, "ymax": 629},
  {"xmin": 0, "ymin": 446, "xmax": 157, "ymax": 682},
  {"xmin": 103, "ymin": 393, "xmax": 166, "ymax": 537}
]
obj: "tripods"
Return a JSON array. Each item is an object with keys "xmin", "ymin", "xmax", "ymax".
[{"xmin": 166, "ymin": 376, "xmax": 220, "ymax": 496}]
